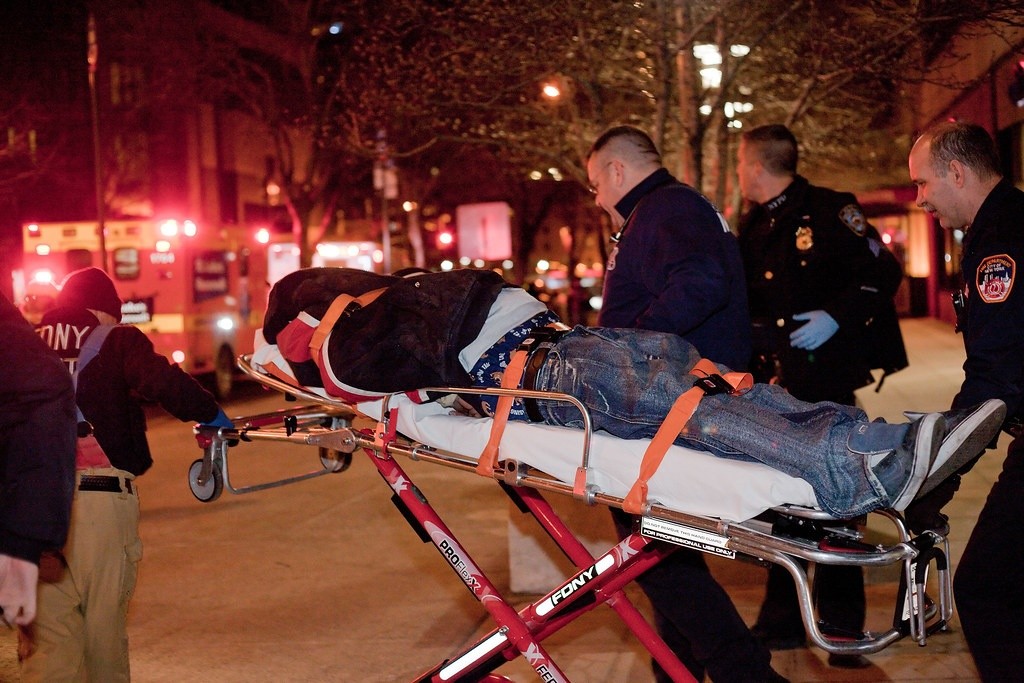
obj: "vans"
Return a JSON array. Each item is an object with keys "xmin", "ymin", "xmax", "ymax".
[{"xmin": 265, "ymin": 239, "xmax": 405, "ymax": 313}]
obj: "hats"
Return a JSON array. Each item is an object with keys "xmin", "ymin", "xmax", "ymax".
[{"xmin": 58, "ymin": 267, "xmax": 122, "ymax": 324}]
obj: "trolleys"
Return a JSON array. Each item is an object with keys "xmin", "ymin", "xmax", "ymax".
[{"xmin": 185, "ymin": 352, "xmax": 956, "ymax": 683}]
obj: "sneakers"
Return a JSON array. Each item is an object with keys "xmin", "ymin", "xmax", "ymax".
[
  {"xmin": 847, "ymin": 412, "xmax": 945, "ymax": 512},
  {"xmin": 903, "ymin": 398, "xmax": 1007, "ymax": 501}
]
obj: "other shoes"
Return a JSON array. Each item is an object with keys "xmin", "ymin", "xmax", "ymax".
[{"xmin": 828, "ymin": 652, "xmax": 861, "ymax": 668}]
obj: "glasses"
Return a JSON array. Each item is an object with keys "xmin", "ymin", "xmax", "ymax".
[
  {"xmin": 23, "ymin": 295, "xmax": 41, "ymax": 303},
  {"xmin": 587, "ymin": 161, "xmax": 624, "ymax": 194}
]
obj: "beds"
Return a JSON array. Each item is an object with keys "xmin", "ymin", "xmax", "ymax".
[{"xmin": 191, "ymin": 329, "xmax": 957, "ymax": 683}]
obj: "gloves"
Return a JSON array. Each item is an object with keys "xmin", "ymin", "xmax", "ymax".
[
  {"xmin": 202, "ymin": 407, "xmax": 239, "ymax": 448},
  {"xmin": 789, "ymin": 310, "xmax": 839, "ymax": 350},
  {"xmin": 905, "ymin": 475, "xmax": 961, "ymax": 532}
]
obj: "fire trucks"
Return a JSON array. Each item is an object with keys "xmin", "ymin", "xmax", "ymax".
[{"xmin": 12, "ymin": 216, "xmax": 272, "ymax": 402}]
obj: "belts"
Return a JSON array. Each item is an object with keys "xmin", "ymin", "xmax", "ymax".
[
  {"xmin": 78, "ymin": 474, "xmax": 133, "ymax": 494},
  {"xmin": 522, "ymin": 330, "xmax": 569, "ymax": 422}
]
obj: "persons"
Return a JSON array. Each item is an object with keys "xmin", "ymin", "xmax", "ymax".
[
  {"xmin": 903, "ymin": 123, "xmax": 1024, "ymax": 683},
  {"xmin": 0, "ymin": 266, "xmax": 239, "ymax": 683},
  {"xmin": 588, "ymin": 122, "xmax": 911, "ymax": 683},
  {"xmin": 263, "ymin": 268, "xmax": 1007, "ymax": 519}
]
